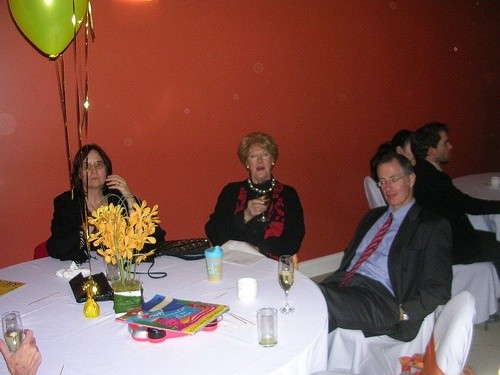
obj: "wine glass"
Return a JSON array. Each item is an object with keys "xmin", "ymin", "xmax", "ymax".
[
  {"xmin": 256, "ymin": 193, "xmax": 269, "ymax": 222},
  {"xmin": 278, "ymin": 255, "xmax": 295, "ymax": 313}
]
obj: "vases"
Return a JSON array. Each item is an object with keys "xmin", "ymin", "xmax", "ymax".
[{"xmin": 111, "ymin": 281, "xmax": 142, "ymax": 314}]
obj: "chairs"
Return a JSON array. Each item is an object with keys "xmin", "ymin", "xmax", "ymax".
[
  {"xmin": 363, "ymin": 176, "xmax": 500, "ymax": 328},
  {"xmin": 323, "ymin": 290, "xmax": 474, "ymax": 375}
]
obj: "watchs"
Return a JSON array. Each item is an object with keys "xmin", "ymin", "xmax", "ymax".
[{"xmin": 403, "ymin": 312, "xmax": 408, "ymax": 321}]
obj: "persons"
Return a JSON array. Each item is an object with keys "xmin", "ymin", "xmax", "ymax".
[
  {"xmin": 319, "ymin": 152, "xmax": 453, "ymax": 343},
  {"xmin": 46, "ymin": 143, "xmax": 139, "ymax": 261},
  {"xmin": 205, "ymin": 130, "xmax": 305, "ymax": 265},
  {"xmin": 370, "ymin": 121, "xmax": 500, "ymax": 279},
  {"xmin": 0, "ymin": 328, "xmax": 42, "ymax": 375}
]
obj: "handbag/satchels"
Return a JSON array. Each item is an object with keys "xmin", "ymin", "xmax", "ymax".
[{"xmin": 158, "ymin": 238, "xmax": 211, "ymax": 261}]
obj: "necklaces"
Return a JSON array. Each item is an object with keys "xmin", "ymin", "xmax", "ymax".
[{"xmin": 249, "ymin": 177, "xmax": 275, "ymax": 193}]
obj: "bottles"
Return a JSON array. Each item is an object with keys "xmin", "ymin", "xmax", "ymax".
[{"xmin": 204, "ymin": 245, "xmax": 223, "ymax": 281}]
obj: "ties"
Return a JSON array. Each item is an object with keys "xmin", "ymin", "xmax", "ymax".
[{"xmin": 339, "ymin": 212, "xmax": 394, "ymax": 289}]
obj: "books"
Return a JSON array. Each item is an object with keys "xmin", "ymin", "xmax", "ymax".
[{"xmin": 115, "ymin": 293, "xmax": 230, "ymax": 335}]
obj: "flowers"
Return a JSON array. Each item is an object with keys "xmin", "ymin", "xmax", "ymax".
[{"xmin": 88, "ymin": 199, "xmax": 161, "ymax": 286}]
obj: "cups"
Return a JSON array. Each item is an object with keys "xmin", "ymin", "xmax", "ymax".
[
  {"xmin": 103, "ymin": 257, "xmax": 120, "ymax": 280},
  {"xmin": 1, "ymin": 311, "xmax": 24, "ymax": 352},
  {"xmin": 491, "ymin": 176, "xmax": 500, "ymax": 188},
  {"xmin": 238, "ymin": 278, "xmax": 258, "ymax": 299},
  {"xmin": 256, "ymin": 307, "xmax": 278, "ymax": 348}
]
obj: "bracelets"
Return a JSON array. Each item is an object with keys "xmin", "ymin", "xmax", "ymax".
[{"xmin": 245, "ymin": 217, "xmax": 248, "ymax": 223}]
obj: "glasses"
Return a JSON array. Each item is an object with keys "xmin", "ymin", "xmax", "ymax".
[
  {"xmin": 377, "ymin": 173, "xmax": 409, "ymax": 188},
  {"xmin": 247, "ymin": 150, "xmax": 272, "ymax": 161},
  {"xmin": 79, "ymin": 161, "xmax": 105, "ymax": 169}
]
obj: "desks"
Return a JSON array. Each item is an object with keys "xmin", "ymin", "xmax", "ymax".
[
  {"xmin": 0, "ymin": 249, "xmax": 326, "ymax": 375},
  {"xmin": 453, "ymin": 173, "xmax": 500, "ymax": 241}
]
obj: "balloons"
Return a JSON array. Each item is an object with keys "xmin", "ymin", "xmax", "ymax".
[{"xmin": 8, "ymin": 0, "xmax": 88, "ymax": 57}]
